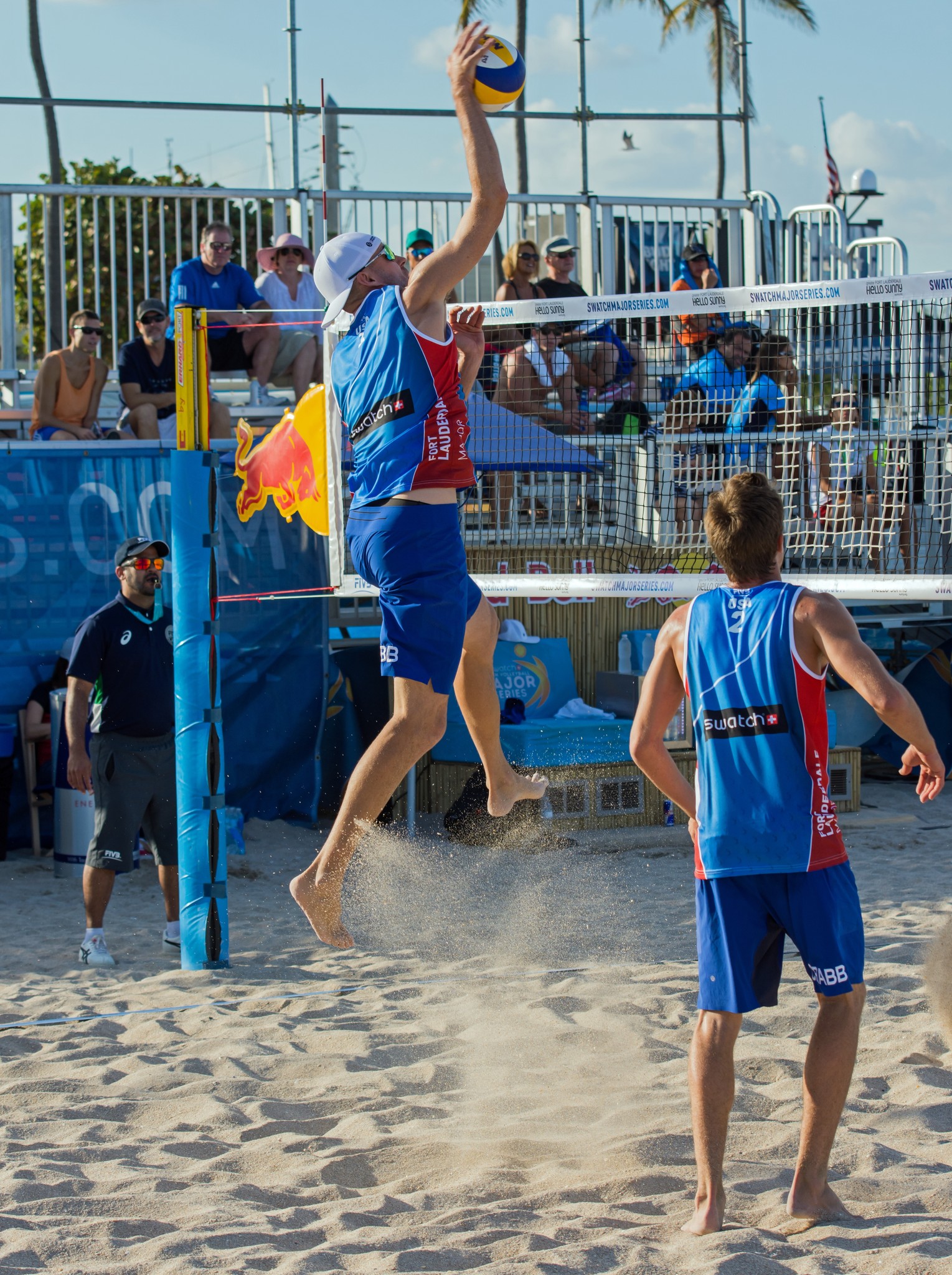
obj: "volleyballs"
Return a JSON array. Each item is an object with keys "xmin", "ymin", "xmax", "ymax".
[{"xmin": 470, "ymin": 34, "xmax": 526, "ymax": 113}]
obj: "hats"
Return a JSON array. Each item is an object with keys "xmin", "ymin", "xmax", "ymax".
[
  {"xmin": 137, "ymin": 298, "xmax": 167, "ymax": 321},
  {"xmin": 681, "ymin": 243, "xmax": 712, "ymax": 260},
  {"xmin": 534, "ymin": 322, "xmax": 564, "ymax": 331},
  {"xmin": 256, "ymin": 233, "xmax": 315, "ymax": 272},
  {"xmin": 540, "ymin": 236, "xmax": 581, "ymax": 255},
  {"xmin": 406, "ymin": 228, "xmax": 434, "ymax": 250},
  {"xmin": 314, "ymin": 232, "xmax": 382, "ymax": 328},
  {"xmin": 114, "ymin": 536, "xmax": 169, "ymax": 564}
]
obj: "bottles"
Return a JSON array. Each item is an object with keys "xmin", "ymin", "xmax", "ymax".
[
  {"xmin": 618, "ymin": 633, "xmax": 632, "ymax": 674},
  {"xmin": 641, "ymin": 632, "xmax": 655, "ymax": 673},
  {"xmin": 249, "ymin": 376, "xmax": 263, "ymax": 407},
  {"xmin": 540, "ymin": 797, "xmax": 553, "ymax": 834},
  {"xmin": 577, "ymin": 390, "xmax": 588, "ymax": 432}
]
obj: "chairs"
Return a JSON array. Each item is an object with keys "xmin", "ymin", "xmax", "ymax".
[{"xmin": 18, "ymin": 711, "xmax": 53, "ymax": 857}]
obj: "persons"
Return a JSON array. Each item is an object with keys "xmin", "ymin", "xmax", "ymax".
[
  {"xmin": 288, "ymin": 20, "xmax": 547, "ymax": 947},
  {"xmin": 628, "ymin": 474, "xmax": 946, "ymax": 1234},
  {"xmin": 165, "ymin": 221, "xmax": 290, "ymax": 410},
  {"xmin": 28, "ymin": 309, "xmax": 136, "ymax": 440},
  {"xmin": 24, "ymin": 656, "xmax": 68, "ymax": 858},
  {"xmin": 404, "ymin": 229, "xmax": 459, "ymax": 304},
  {"xmin": 254, "ymin": 233, "xmax": 325, "ymax": 408},
  {"xmin": 68, "ymin": 538, "xmax": 180, "ymax": 966},
  {"xmin": 490, "ymin": 234, "xmax": 921, "ymax": 574},
  {"xmin": 117, "ymin": 299, "xmax": 232, "ymax": 439}
]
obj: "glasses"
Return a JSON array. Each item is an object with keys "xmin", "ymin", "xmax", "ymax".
[
  {"xmin": 407, "ymin": 248, "xmax": 434, "ymax": 258},
  {"xmin": 120, "ymin": 557, "xmax": 164, "ymax": 571},
  {"xmin": 209, "ymin": 241, "xmax": 233, "ymax": 251},
  {"xmin": 779, "ymin": 351, "xmax": 794, "ymax": 356},
  {"xmin": 73, "ymin": 325, "xmax": 104, "ymax": 336},
  {"xmin": 278, "ymin": 248, "xmax": 302, "ymax": 257},
  {"xmin": 518, "ymin": 252, "xmax": 540, "ymax": 261},
  {"xmin": 346, "ymin": 244, "xmax": 395, "ymax": 281},
  {"xmin": 835, "ymin": 401, "xmax": 857, "ymax": 408},
  {"xmin": 141, "ymin": 314, "xmax": 166, "ymax": 325},
  {"xmin": 549, "ymin": 252, "xmax": 577, "ymax": 258},
  {"xmin": 540, "ymin": 327, "xmax": 562, "ymax": 336}
]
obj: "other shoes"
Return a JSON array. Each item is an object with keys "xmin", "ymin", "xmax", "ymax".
[
  {"xmin": 517, "ymin": 502, "xmax": 555, "ymax": 520},
  {"xmin": 576, "ymin": 498, "xmax": 609, "ymax": 514}
]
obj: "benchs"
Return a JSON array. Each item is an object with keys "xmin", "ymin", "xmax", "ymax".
[
  {"xmin": 429, "ymin": 638, "xmax": 656, "ymax": 828},
  {"xmin": 14, "ymin": 366, "xmax": 689, "ymax": 539},
  {"xmin": 618, "ymin": 630, "xmax": 861, "ymax": 813}
]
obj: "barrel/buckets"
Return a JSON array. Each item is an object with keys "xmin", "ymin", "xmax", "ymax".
[{"xmin": 50, "ymin": 687, "xmax": 140, "ymax": 880}]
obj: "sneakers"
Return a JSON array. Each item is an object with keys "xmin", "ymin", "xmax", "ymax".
[
  {"xmin": 574, "ymin": 383, "xmax": 596, "ymax": 401},
  {"xmin": 597, "ymin": 380, "xmax": 635, "ymax": 401},
  {"xmin": 77, "ymin": 935, "xmax": 115, "ymax": 967},
  {"xmin": 161, "ymin": 930, "xmax": 181, "ymax": 954}
]
